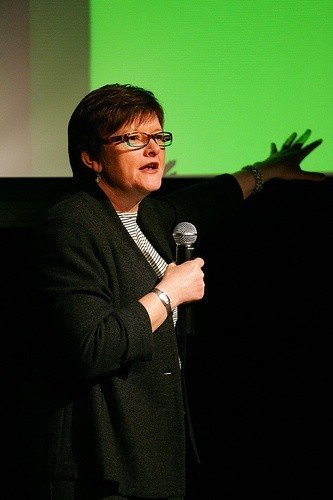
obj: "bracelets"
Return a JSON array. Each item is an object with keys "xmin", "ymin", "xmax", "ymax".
[{"xmin": 243, "ymin": 166, "xmax": 264, "ymax": 196}]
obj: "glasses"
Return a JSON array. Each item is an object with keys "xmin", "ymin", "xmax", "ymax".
[{"xmin": 100, "ymin": 132, "xmax": 173, "ymax": 147}]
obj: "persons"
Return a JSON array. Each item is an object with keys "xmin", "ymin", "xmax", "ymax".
[{"xmin": 36, "ymin": 85, "xmax": 325, "ymax": 500}]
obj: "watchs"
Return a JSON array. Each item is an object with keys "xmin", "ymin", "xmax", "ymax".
[{"xmin": 149, "ymin": 288, "xmax": 172, "ymax": 316}]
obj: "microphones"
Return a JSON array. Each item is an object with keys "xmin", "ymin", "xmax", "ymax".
[{"xmin": 173, "ymin": 222, "xmax": 197, "ymax": 334}]
